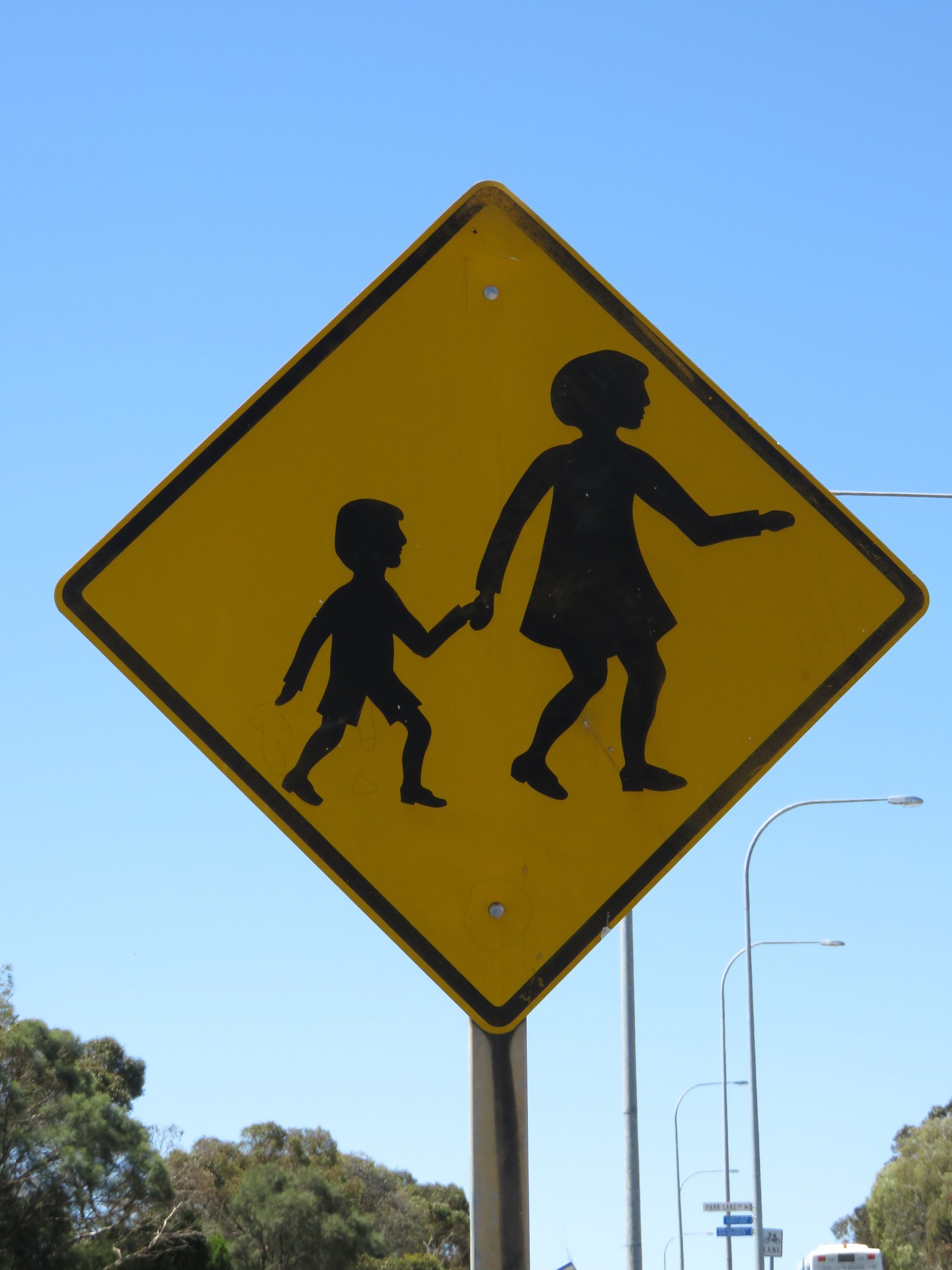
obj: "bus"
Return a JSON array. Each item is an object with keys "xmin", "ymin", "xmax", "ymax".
[{"xmin": 797, "ymin": 1243, "xmax": 883, "ymax": 1270}]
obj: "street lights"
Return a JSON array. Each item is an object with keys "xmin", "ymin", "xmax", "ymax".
[
  {"xmin": 663, "ymin": 1229, "xmax": 718, "ymax": 1270},
  {"xmin": 719, "ymin": 938, "xmax": 846, "ymax": 1270},
  {"xmin": 679, "ymin": 1166, "xmax": 738, "ymax": 1270},
  {"xmin": 674, "ymin": 1079, "xmax": 751, "ymax": 1270},
  {"xmin": 742, "ymin": 795, "xmax": 926, "ymax": 1270}
]
